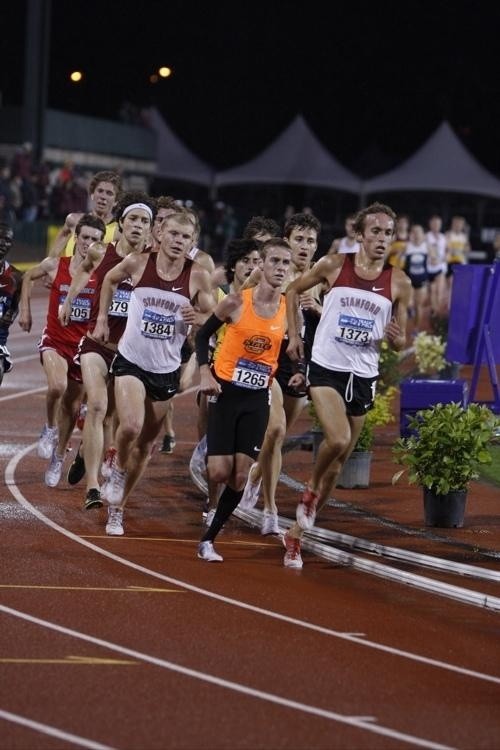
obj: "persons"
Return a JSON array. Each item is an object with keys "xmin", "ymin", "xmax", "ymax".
[{"xmin": 3, "ymin": 158, "xmax": 469, "ymax": 570}]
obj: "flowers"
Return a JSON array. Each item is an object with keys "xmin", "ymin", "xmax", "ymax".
[
  {"xmin": 413, "ymin": 330, "xmax": 448, "ymax": 373},
  {"xmin": 379, "ymin": 341, "xmax": 399, "ymax": 377}
]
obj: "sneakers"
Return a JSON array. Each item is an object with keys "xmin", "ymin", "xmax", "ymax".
[
  {"xmin": 196, "ymin": 463, "xmax": 321, "ymax": 568},
  {"xmin": 38, "ymin": 403, "xmax": 129, "ymax": 536},
  {"xmin": 160, "ymin": 434, "xmax": 175, "ymax": 453}
]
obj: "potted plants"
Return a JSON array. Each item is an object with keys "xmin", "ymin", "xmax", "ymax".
[
  {"xmin": 310, "ymin": 400, "xmax": 326, "ymax": 461},
  {"xmin": 392, "ymin": 401, "xmax": 496, "ymax": 528},
  {"xmin": 337, "ymin": 385, "xmax": 400, "ymax": 488}
]
